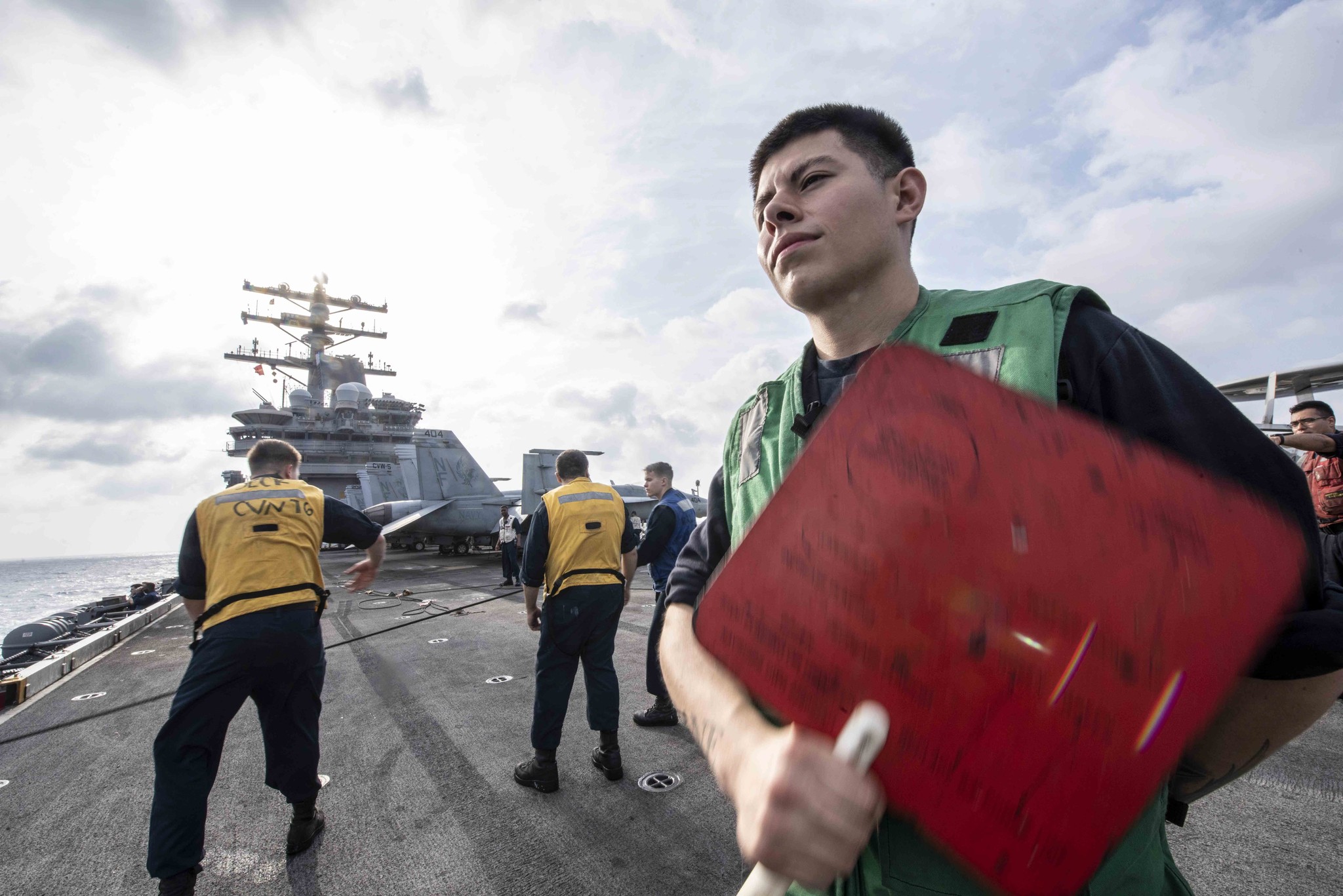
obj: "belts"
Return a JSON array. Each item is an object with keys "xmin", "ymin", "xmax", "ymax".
[{"xmin": 1318, "ymin": 521, "xmax": 1343, "ymax": 535}]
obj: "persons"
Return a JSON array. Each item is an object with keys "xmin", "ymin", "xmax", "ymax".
[
  {"xmin": 514, "ymin": 450, "xmax": 637, "ymax": 793},
  {"xmin": 495, "ymin": 505, "xmax": 522, "ymax": 586},
  {"xmin": 128, "ymin": 581, "xmax": 161, "ymax": 617},
  {"xmin": 1269, "ymin": 401, "xmax": 1343, "ymax": 704},
  {"xmin": 658, "ymin": 105, "xmax": 1343, "ymax": 896},
  {"xmin": 630, "ymin": 511, "xmax": 641, "ymax": 548},
  {"xmin": 633, "ymin": 462, "xmax": 697, "ymax": 728},
  {"xmin": 145, "ymin": 439, "xmax": 386, "ymax": 896}
]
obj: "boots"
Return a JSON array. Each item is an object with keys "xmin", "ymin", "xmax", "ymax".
[
  {"xmin": 514, "ymin": 750, "xmax": 558, "ymax": 793},
  {"xmin": 515, "ymin": 575, "xmax": 522, "ymax": 586},
  {"xmin": 499, "ymin": 577, "xmax": 513, "ymax": 586},
  {"xmin": 633, "ymin": 697, "xmax": 678, "ymax": 727},
  {"xmin": 286, "ymin": 804, "xmax": 325, "ymax": 850},
  {"xmin": 591, "ymin": 730, "xmax": 623, "ymax": 780}
]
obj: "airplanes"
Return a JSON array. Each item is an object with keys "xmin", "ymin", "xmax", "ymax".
[
  {"xmin": 359, "ymin": 493, "xmax": 523, "ymax": 556},
  {"xmin": 533, "ymin": 479, "xmax": 708, "ymax": 524}
]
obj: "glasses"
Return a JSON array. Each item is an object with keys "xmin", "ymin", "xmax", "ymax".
[{"xmin": 1287, "ymin": 417, "xmax": 1329, "ymax": 429}]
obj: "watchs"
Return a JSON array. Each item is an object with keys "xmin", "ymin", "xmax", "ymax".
[{"xmin": 1270, "ymin": 433, "xmax": 1284, "ymax": 445}]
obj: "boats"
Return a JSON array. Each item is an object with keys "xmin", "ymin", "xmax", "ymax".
[{"xmin": 218, "ymin": 268, "xmax": 524, "ymax": 556}]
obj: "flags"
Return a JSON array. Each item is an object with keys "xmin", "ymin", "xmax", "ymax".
[
  {"xmin": 269, "ymin": 299, "xmax": 275, "ymax": 305},
  {"xmin": 253, "ymin": 364, "xmax": 264, "ymax": 376}
]
